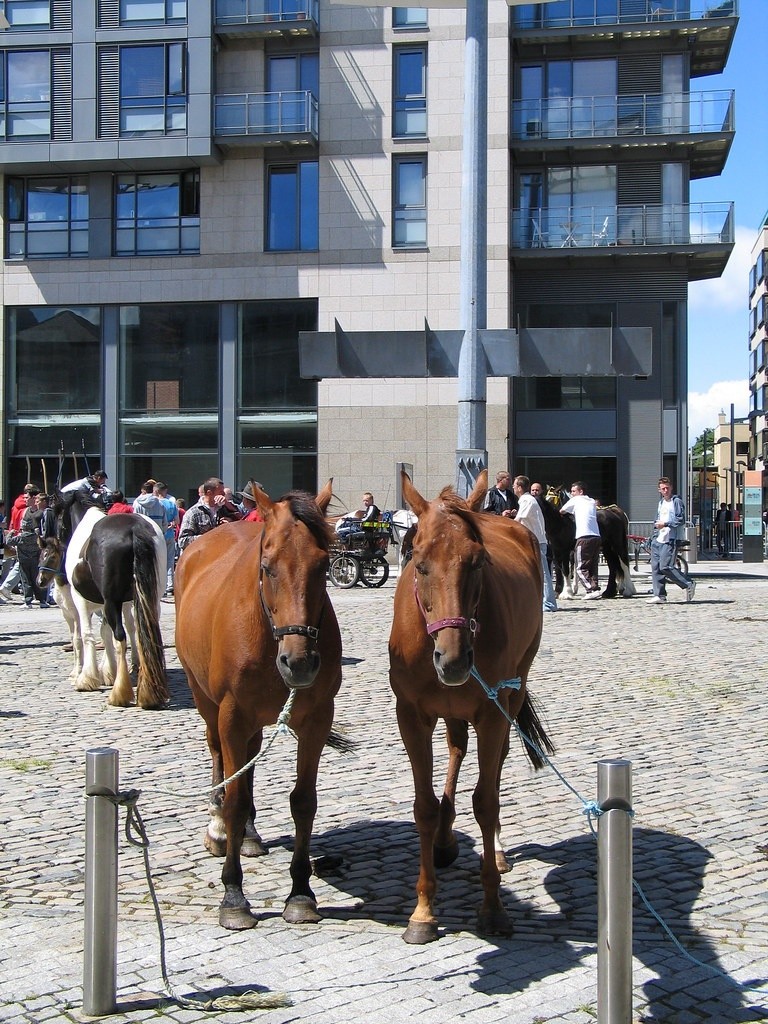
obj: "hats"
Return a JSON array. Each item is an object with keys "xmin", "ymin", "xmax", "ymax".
[
  {"xmin": 19, "ymin": 519, "xmax": 33, "ymax": 530},
  {"xmin": 24, "ymin": 483, "xmax": 41, "ymax": 493},
  {"xmin": 236, "ymin": 481, "xmax": 269, "ymax": 502}
]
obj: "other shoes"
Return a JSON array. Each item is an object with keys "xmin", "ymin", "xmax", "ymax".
[
  {"xmin": 722, "ymin": 553, "xmax": 729, "ymax": 558},
  {"xmin": 718, "ymin": 547, "xmax": 723, "ymax": 554},
  {"xmin": 0, "ymin": 569, "xmax": 176, "ymax": 610}
]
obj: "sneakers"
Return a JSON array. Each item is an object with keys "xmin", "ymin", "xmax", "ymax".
[
  {"xmin": 645, "ymin": 596, "xmax": 667, "ymax": 604},
  {"xmin": 583, "ymin": 590, "xmax": 602, "ymax": 600},
  {"xmin": 686, "ymin": 580, "xmax": 696, "ymax": 602}
]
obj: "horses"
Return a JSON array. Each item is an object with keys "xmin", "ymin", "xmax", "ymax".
[
  {"xmin": 387, "ymin": 466, "xmax": 556, "ymax": 945},
  {"xmin": 532, "ymin": 484, "xmax": 636, "ymax": 599},
  {"xmin": 172, "ymin": 477, "xmax": 342, "ymax": 931},
  {"xmin": 36, "ymin": 485, "xmax": 167, "ymax": 711}
]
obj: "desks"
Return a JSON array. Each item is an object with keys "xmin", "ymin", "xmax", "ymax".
[{"xmin": 560, "ymin": 221, "xmax": 581, "ymax": 247}]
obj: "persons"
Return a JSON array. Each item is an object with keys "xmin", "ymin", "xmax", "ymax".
[
  {"xmin": 334, "ymin": 491, "xmax": 379, "ymax": 540},
  {"xmin": 559, "ymin": 481, "xmax": 602, "ymax": 600},
  {"xmin": 484, "ymin": 471, "xmax": 520, "ymax": 522},
  {"xmin": 132, "ymin": 479, "xmax": 188, "ymax": 597},
  {"xmin": 512, "ymin": 477, "xmax": 558, "ymax": 612},
  {"xmin": 529, "ymin": 483, "xmax": 553, "ymax": 576},
  {"xmin": 178, "ymin": 478, "xmax": 265, "ymax": 550},
  {"xmin": 0, "ymin": 483, "xmax": 58, "ymax": 609},
  {"xmin": 78, "ymin": 469, "xmax": 108, "ymax": 501},
  {"xmin": 645, "ymin": 476, "xmax": 696, "ymax": 605},
  {"xmin": 727, "ymin": 504, "xmax": 740, "ymax": 549},
  {"xmin": 715, "ymin": 504, "xmax": 732, "ymax": 557},
  {"xmin": 108, "ymin": 490, "xmax": 134, "ymax": 515}
]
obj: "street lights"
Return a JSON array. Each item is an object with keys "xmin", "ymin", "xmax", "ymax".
[
  {"xmin": 713, "ymin": 472, "xmax": 720, "ymax": 519},
  {"xmin": 701, "ymin": 430, "xmax": 731, "ymax": 550},
  {"xmin": 737, "ymin": 461, "xmax": 747, "ymax": 515},
  {"xmin": 727, "ymin": 403, "xmax": 765, "ymax": 559},
  {"xmin": 723, "ymin": 466, "xmax": 731, "ymax": 504},
  {"xmin": 698, "ymin": 467, "xmax": 713, "ymax": 548}
]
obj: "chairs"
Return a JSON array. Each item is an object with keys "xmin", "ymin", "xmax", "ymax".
[
  {"xmin": 593, "ymin": 217, "xmax": 611, "ymax": 247},
  {"xmin": 531, "ymin": 218, "xmax": 550, "ymax": 248},
  {"xmin": 650, "ymin": 0, "xmax": 674, "ymax": 21}
]
